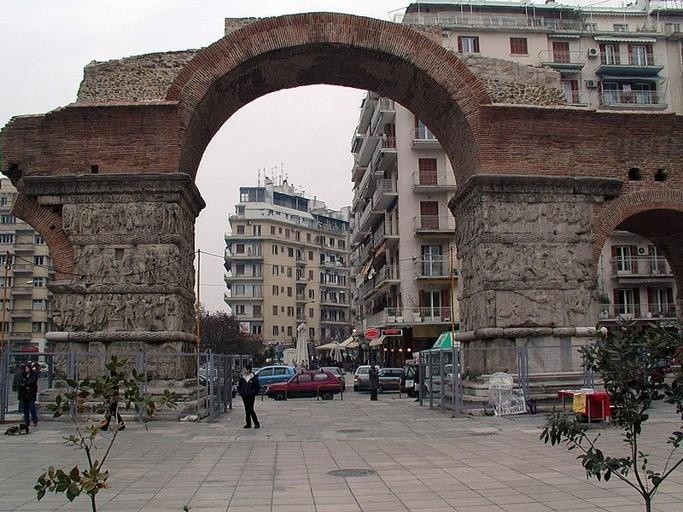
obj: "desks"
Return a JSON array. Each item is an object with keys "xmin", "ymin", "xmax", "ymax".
[{"xmin": 556, "ymin": 390, "xmax": 605, "ymax": 426}]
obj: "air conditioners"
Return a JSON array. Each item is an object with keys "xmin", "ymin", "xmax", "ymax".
[
  {"xmin": 586, "ymin": 79, "xmax": 598, "ymax": 89},
  {"xmin": 637, "ymin": 247, "xmax": 649, "ymax": 255},
  {"xmin": 587, "ymin": 47, "xmax": 599, "ymax": 59}
]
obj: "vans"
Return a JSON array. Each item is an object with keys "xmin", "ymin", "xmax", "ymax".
[{"xmin": 406, "ymin": 348, "xmax": 460, "ymax": 398}]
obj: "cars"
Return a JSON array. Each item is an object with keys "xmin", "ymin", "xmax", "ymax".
[
  {"xmin": 29, "ymin": 361, "xmax": 50, "ymax": 378},
  {"xmin": 199, "ymin": 361, "xmax": 237, "ymax": 398},
  {"xmin": 9, "ymin": 361, "xmax": 26, "ymax": 374},
  {"xmin": 354, "ymin": 365, "xmax": 403, "ymax": 392},
  {"xmin": 240, "ymin": 366, "xmax": 346, "ymax": 400}
]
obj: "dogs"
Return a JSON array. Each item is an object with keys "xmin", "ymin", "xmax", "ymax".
[{"xmin": 4, "ymin": 423, "xmax": 29, "ymax": 436}]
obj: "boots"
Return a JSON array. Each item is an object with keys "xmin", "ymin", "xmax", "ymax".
[
  {"xmin": 99, "ymin": 421, "xmax": 125, "ymax": 431},
  {"xmin": 26, "ymin": 422, "xmax": 39, "ymax": 427},
  {"xmin": 244, "ymin": 423, "xmax": 260, "ymax": 428}
]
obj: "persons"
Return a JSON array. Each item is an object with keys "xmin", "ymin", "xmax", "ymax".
[
  {"xmin": 96, "ymin": 368, "xmax": 126, "ymax": 431},
  {"xmin": 12, "ymin": 362, "xmax": 41, "ymax": 427},
  {"xmin": 368, "ymin": 360, "xmax": 380, "ymax": 401},
  {"xmin": 413, "ymin": 363, "xmax": 424, "ymax": 402},
  {"xmin": 237, "ymin": 365, "xmax": 260, "ymax": 429},
  {"xmin": 274, "ymin": 349, "xmax": 385, "ymax": 374}
]
